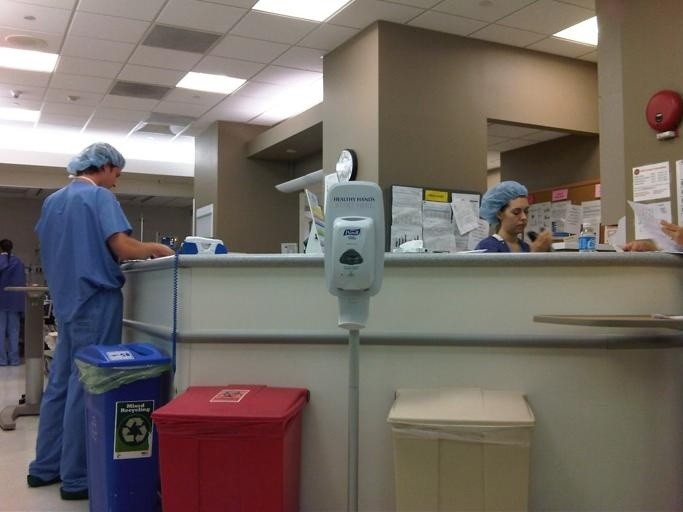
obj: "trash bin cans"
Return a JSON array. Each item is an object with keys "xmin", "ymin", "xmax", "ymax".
[
  {"xmin": 151, "ymin": 384, "xmax": 308, "ymax": 511},
  {"xmin": 76, "ymin": 342, "xmax": 174, "ymax": 510},
  {"xmin": 385, "ymin": 386, "xmax": 534, "ymax": 510}
]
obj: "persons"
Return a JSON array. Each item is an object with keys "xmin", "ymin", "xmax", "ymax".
[
  {"xmin": 0, "ymin": 238, "xmax": 24, "ymax": 368},
  {"xmin": 621, "ymin": 218, "xmax": 682, "ymax": 252},
  {"xmin": 24, "ymin": 140, "xmax": 177, "ymax": 501},
  {"xmin": 473, "ymin": 180, "xmax": 554, "ymax": 252}
]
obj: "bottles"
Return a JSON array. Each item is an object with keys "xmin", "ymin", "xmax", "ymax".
[{"xmin": 578, "ymin": 222, "xmax": 597, "ymax": 253}]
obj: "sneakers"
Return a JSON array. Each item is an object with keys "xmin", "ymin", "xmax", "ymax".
[
  {"xmin": 58, "ymin": 486, "xmax": 88, "ymax": 500},
  {"xmin": 26, "ymin": 473, "xmax": 61, "ymax": 488}
]
